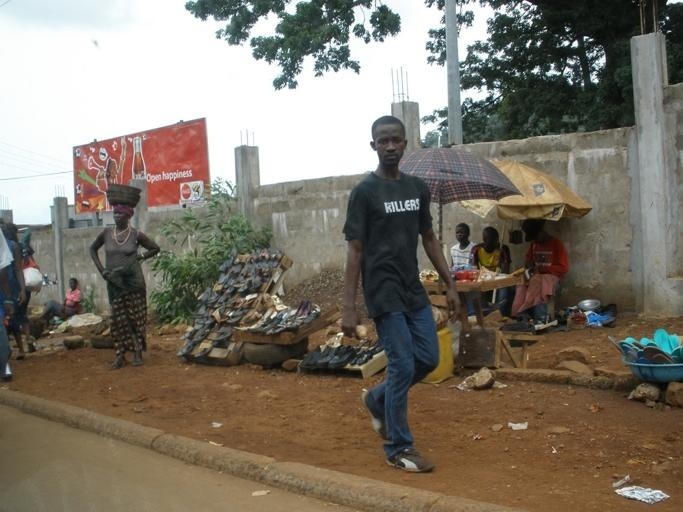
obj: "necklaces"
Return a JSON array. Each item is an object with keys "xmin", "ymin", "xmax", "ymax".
[{"xmin": 113, "ymin": 225, "xmax": 132, "ymax": 245}]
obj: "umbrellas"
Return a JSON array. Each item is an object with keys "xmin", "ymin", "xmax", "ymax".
[
  {"xmin": 398, "ymin": 137, "xmax": 522, "ymax": 252},
  {"xmin": 463, "ymin": 158, "xmax": 592, "ymax": 243}
]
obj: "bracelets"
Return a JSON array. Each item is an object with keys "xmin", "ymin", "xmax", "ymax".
[{"xmin": 140, "ymin": 252, "xmax": 146, "ymax": 260}]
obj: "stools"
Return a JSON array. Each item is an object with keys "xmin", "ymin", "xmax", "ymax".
[
  {"xmin": 546, "ymin": 284, "xmax": 563, "ymax": 321},
  {"xmin": 457, "ymin": 329, "xmax": 546, "ymax": 371}
]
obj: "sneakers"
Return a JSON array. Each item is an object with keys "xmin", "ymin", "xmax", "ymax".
[
  {"xmin": 362, "ymin": 391, "xmax": 387, "ymax": 440},
  {"xmin": 386, "ymin": 447, "xmax": 433, "ymax": 472}
]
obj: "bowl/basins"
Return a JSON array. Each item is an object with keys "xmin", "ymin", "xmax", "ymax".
[
  {"xmin": 105, "ymin": 191, "xmax": 140, "ymax": 208},
  {"xmin": 104, "ymin": 183, "xmax": 141, "ymax": 197},
  {"xmin": 577, "ymin": 299, "xmax": 601, "ymax": 313},
  {"xmin": 620, "ymin": 357, "xmax": 683, "ymax": 384},
  {"xmin": 454, "ymin": 269, "xmax": 479, "ymax": 280}
]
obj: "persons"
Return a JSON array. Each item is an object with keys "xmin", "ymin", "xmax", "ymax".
[
  {"xmin": 0, "ymin": 217, "xmax": 44, "ymax": 382},
  {"xmin": 341, "ymin": 113, "xmax": 463, "ymax": 473},
  {"xmin": 449, "ymin": 221, "xmax": 478, "ymax": 327},
  {"xmin": 468, "ymin": 225, "xmax": 513, "ymax": 327},
  {"xmin": 44, "ymin": 277, "xmax": 83, "ymax": 320},
  {"xmin": 516, "ymin": 218, "xmax": 570, "ymax": 332},
  {"xmin": 90, "ymin": 205, "xmax": 159, "ymax": 368}
]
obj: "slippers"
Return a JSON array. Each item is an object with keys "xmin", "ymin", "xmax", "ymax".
[
  {"xmin": 179, "ymin": 252, "xmax": 383, "ymax": 369},
  {"xmin": 620, "ymin": 328, "xmax": 683, "ymax": 364}
]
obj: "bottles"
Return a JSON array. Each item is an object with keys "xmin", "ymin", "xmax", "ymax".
[{"xmin": 131, "ymin": 136, "xmax": 146, "ymax": 180}]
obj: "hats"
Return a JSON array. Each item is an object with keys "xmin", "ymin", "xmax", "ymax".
[{"xmin": 114, "ymin": 204, "xmax": 134, "ymax": 217}]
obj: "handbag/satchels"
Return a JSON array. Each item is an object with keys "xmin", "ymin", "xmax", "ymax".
[{"xmin": 23, "ymin": 268, "xmax": 42, "ymax": 292}]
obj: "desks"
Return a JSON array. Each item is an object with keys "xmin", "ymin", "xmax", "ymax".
[{"xmin": 411, "ymin": 272, "xmax": 524, "ymax": 332}]
